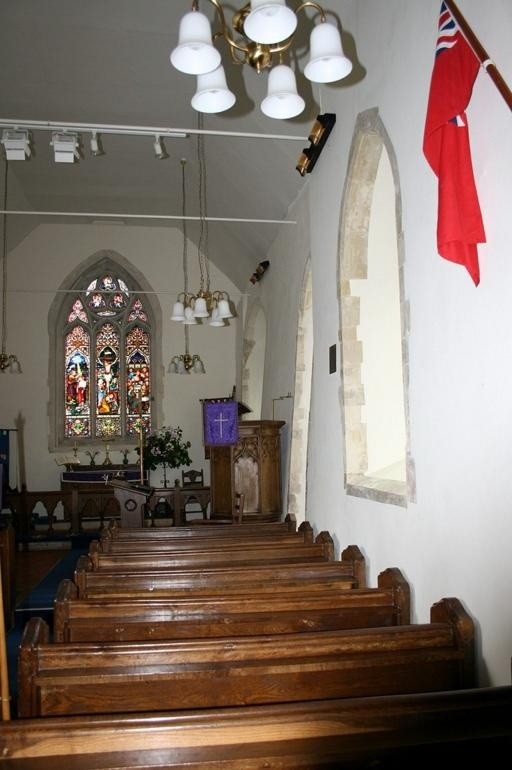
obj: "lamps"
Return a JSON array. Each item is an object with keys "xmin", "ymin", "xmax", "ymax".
[
  {"xmin": 170, "ymin": 0, "xmax": 353, "ymax": 119},
  {"xmin": 166, "ymin": 158, "xmax": 206, "ymax": 375},
  {"xmin": 0, "ymin": 119, "xmax": 187, "ymax": 163},
  {"xmin": 295, "ymin": 113, "xmax": 336, "ymax": 176},
  {"xmin": 249, "ymin": 261, "xmax": 269, "ymax": 285},
  {"xmin": 170, "ymin": 112, "xmax": 234, "ymax": 327},
  {"xmin": 0, "ymin": 156, "xmax": 23, "ymax": 374}
]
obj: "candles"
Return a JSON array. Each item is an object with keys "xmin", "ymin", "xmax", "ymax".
[{"xmin": 75, "ymin": 441, "xmax": 76, "ymax": 450}]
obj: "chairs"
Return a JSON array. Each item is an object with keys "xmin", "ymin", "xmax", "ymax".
[
  {"xmin": 181, "ymin": 468, "xmax": 208, "ymax": 521},
  {"xmin": 190, "ymin": 492, "xmax": 245, "ymax": 525}
]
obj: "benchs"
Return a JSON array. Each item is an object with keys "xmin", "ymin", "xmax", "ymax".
[{"xmin": 0, "ymin": 513, "xmax": 512, "ymax": 770}]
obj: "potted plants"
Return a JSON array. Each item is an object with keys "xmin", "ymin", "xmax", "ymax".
[{"xmin": 134, "ymin": 426, "xmax": 192, "ymax": 518}]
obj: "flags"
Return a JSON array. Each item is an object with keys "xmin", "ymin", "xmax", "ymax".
[{"xmin": 425, "ymin": 2, "xmax": 481, "ymax": 286}]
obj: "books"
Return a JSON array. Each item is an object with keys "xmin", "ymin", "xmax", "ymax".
[{"xmin": 55, "ymin": 455, "xmax": 81, "ymax": 465}]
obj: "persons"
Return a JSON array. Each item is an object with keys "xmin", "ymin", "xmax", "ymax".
[{"xmin": 68, "ymin": 355, "xmax": 151, "ymax": 414}]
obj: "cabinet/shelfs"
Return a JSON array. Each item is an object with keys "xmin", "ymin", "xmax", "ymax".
[{"xmin": 60, "ymin": 463, "xmax": 147, "ymax": 522}]
